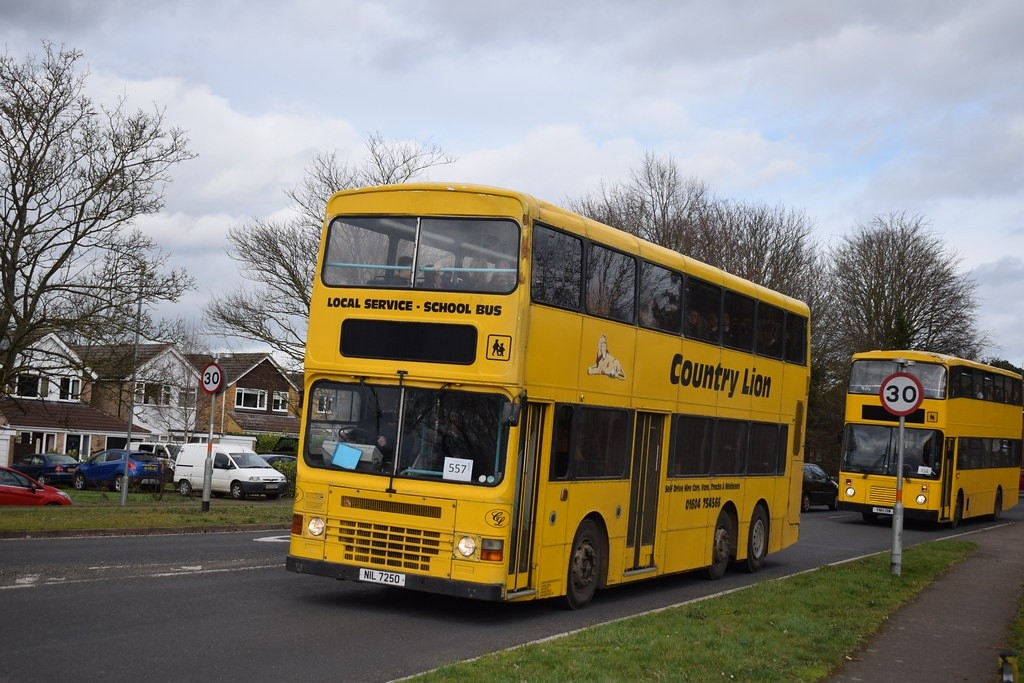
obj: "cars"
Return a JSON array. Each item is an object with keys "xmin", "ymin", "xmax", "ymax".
[
  {"xmin": 0, "ymin": 466, "xmax": 70, "ymax": 506},
  {"xmin": 3, "ymin": 454, "xmax": 82, "ymax": 489},
  {"xmin": 800, "ymin": 463, "xmax": 839, "ymax": 512},
  {"xmin": 259, "ymin": 453, "xmax": 296, "ymax": 470}
]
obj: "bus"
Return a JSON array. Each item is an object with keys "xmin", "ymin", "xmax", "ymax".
[
  {"xmin": 284, "ymin": 183, "xmax": 813, "ymax": 611},
  {"xmin": 838, "ymin": 349, "xmax": 1024, "ymax": 528}
]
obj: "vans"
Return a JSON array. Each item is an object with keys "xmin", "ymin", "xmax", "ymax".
[
  {"xmin": 172, "ymin": 443, "xmax": 286, "ymax": 501},
  {"xmin": 121, "ymin": 442, "xmax": 185, "ymax": 483}
]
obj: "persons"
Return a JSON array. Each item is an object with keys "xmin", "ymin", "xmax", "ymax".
[
  {"xmin": 389, "ymin": 256, "xmax": 793, "ymax": 360},
  {"xmin": 336, "ymin": 395, "xmax": 415, "ymax": 473},
  {"xmin": 857, "ymin": 374, "xmax": 1021, "ymax": 405},
  {"xmin": 960, "ymin": 439, "xmax": 1015, "ymax": 467}
]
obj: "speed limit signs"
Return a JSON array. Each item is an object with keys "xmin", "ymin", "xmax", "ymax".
[
  {"xmin": 879, "ymin": 372, "xmax": 924, "ymax": 417},
  {"xmin": 200, "ymin": 362, "xmax": 224, "ymax": 394}
]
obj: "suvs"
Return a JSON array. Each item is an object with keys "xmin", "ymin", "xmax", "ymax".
[{"xmin": 71, "ymin": 448, "xmax": 163, "ymax": 492}]
